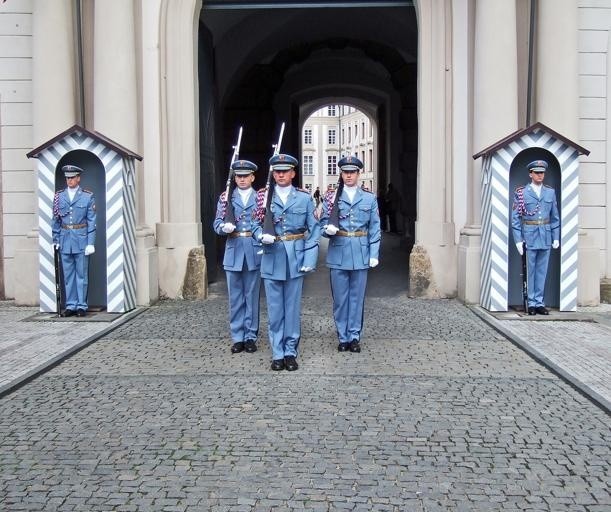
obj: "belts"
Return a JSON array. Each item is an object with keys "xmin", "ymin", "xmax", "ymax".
[
  {"xmin": 522, "ymin": 219, "xmax": 550, "ymax": 225},
  {"xmin": 230, "ymin": 231, "xmax": 252, "ymax": 237},
  {"xmin": 336, "ymin": 230, "xmax": 367, "ymax": 237},
  {"xmin": 61, "ymin": 223, "xmax": 88, "ymax": 229},
  {"xmin": 276, "ymin": 233, "xmax": 304, "ymax": 241}
]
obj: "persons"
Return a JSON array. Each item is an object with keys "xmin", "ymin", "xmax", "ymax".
[
  {"xmin": 361, "ymin": 181, "xmax": 364, "ymax": 190},
  {"xmin": 320, "ymin": 157, "xmax": 381, "ymax": 354},
  {"xmin": 377, "ymin": 191, "xmax": 388, "ymax": 232},
  {"xmin": 52, "ymin": 165, "xmax": 97, "ymax": 318},
  {"xmin": 314, "ymin": 186, "xmax": 322, "ymax": 209},
  {"xmin": 213, "ymin": 160, "xmax": 264, "ymax": 354},
  {"xmin": 512, "ymin": 160, "xmax": 560, "ymax": 314},
  {"xmin": 386, "ymin": 180, "xmax": 399, "ymax": 234},
  {"xmin": 251, "ymin": 155, "xmax": 321, "ymax": 371}
]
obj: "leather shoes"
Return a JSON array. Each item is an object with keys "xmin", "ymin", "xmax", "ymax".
[
  {"xmin": 271, "ymin": 359, "xmax": 285, "ymax": 370},
  {"xmin": 536, "ymin": 305, "xmax": 548, "ymax": 315},
  {"xmin": 528, "ymin": 306, "xmax": 537, "ymax": 315},
  {"xmin": 231, "ymin": 342, "xmax": 244, "ymax": 353},
  {"xmin": 244, "ymin": 339, "xmax": 257, "ymax": 353},
  {"xmin": 337, "ymin": 342, "xmax": 349, "ymax": 351},
  {"xmin": 76, "ymin": 308, "xmax": 86, "ymax": 317},
  {"xmin": 62, "ymin": 309, "xmax": 76, "ymax": 317},
  {"xmin": 285, "ymin": 355, "xmax": 298, "ymax": 371},
  {"xmin": 349, "ymin": 339, "xmax": 361, "ymax": 352}
]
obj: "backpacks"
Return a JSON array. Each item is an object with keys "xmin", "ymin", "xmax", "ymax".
[{"xmin": 314, "ymin": 193, "xmax": 316, "ymax": 198}]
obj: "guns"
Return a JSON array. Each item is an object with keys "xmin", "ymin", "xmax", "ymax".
[
  {"xmin": 522, "ymin": 242, "xmax": 529, "ymax": 313},
  {"xmin": 327, "ymin": 133, "xmax": 358, "ymax": 228},
  {"xmin": 224, "ymin": 127, "xmax": 243, "ymax": 226},
  {"xmin": 54, "ymin": 244, "xmax": 62, "ymax": 317},
  {"xmin": 262, "ymin": 121, "xmax": 285, "ymax": 236}
]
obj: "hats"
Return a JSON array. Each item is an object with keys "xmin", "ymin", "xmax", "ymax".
[
  {"xmin": 337, "ymin": 155, "xmax": 364, "ymax": 171},
  {"xmin": 269, "ymin": 154, "xmax": 299, "ymax": 171},
  {"xmin": 527, "ymin": 160, "xmax": 548, "ymax": 172},
  {"xmin": 61, "ymin": 165, "xmax": 85, "ymax": 178},
  {"xmin": 231, "ymin": 160, "xmax": 258, "ymax": 175}
]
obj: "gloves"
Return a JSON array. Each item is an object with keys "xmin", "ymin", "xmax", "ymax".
[
  {"xmin": 323, "ymin": 224, "xmax": 337, "ymax": 236},
  {"xmin": 52, "ymin": 242, "xmax": 60, "ymax": 250},
  {"xmin": 369, "ymin": 258, "xmax": 379, "ymax": 268},
  {"xmin": 300, "ymin": 266, "xmax": 313, "ymax": 272},
  {"xmin": 516, "ymin": 241, "xmax": 527, "ymax": 255},
  {"xmin": 551, "ymin": 240, "xmax": 559, "ymax": 249},
  {"xmin": 258, "ymin": 233, "xmax": 276, "ymax": 245},
  {"xmin": 84, "ymin": 245, "xmax": 96, "ymax": 256},
  {"xmin": 219, "ymin": 222, "xmax": 236, "ymax": 234}
]
